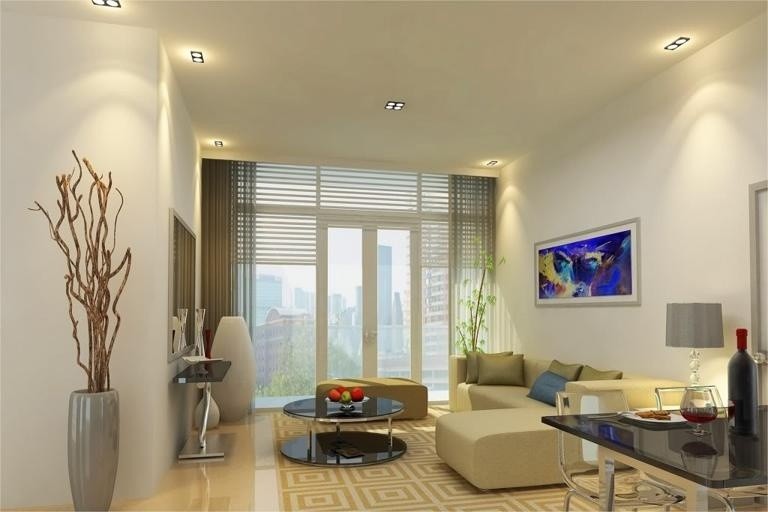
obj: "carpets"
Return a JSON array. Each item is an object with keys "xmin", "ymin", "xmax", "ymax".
[{"xmin": 266, "ymin": 403, "xmax": 767, "ymax": 511}]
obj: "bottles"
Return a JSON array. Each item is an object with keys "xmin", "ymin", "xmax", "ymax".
[
  {"xmin": 195, "ymin": 385, "xmax": 220, "ymax": 431},
  {"xmin": 726, "ymin": 328, "xmax": 759, "ymax": 438},
  {"xmin": 726, "ymin": 439, "xmax": 760, "ymax": 479}
]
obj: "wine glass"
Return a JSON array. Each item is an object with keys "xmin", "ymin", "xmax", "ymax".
[
  {"xmin": 679, "ymin": 386, "xmax": 718, "ymax": 438},
  {"xmin": 678, "ymin": 428, "xmax": 719, "ymax": 478}
]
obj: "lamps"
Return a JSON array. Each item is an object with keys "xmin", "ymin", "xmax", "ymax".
[{"xmin": 663, "ymin": 300, "xmax": 728, "ymax": 388}]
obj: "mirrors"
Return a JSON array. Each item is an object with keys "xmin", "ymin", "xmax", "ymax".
[{"xmin": 166, "ymin": 204, "xmax": 199, "ymax": 368}]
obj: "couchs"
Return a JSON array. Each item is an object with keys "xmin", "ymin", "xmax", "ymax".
[{"xmin": 434, "ymin": 352, "xmax": 695, "ymax": 494}]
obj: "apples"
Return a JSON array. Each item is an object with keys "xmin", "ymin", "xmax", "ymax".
[{"xmin": 328, "ymin": 387, "xmax": 364, "ymax": 402}]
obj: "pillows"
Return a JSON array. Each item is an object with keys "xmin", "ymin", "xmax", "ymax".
[
  {"xmin": 546, "ymin": 358, "xmax": 583, "ymax": 383},
  {"xmin": 525, "ymin": 369, "xmax": 571, "ymax": 409},
  {"xmin": 577, "ymin": 361, "xmax": 624, "ymax": 383},
  {"xmin": 474, "ymin": 351, "xmax": 527, "ymax": 387},
  {"xmin": 462, "ymin": 349, "xmax": 515, "ymax": 385}
]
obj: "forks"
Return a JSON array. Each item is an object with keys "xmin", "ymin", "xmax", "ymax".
[{"xmin": 587, "ymin": 410, "xmax": 633, "ymax": 420}]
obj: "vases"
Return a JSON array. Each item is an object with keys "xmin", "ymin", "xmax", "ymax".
[
  {"xmin": 190, "ymin": 304, "xmax": 208, "ymax": 359},
  {"xmin": 175, "ymin": 307, "xmax": 190, "ymax": 353},
  {"xmin": 192, "ymin": 388, "xmax": 222, "ymax": 428},
  {"xmin": 63, "ymin": 386, "xmax": 122, "ymax": 511}
]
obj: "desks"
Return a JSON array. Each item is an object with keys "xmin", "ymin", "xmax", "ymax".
[
  {"xmin": 170, "ymin": 359, "xmax": 234, "ymax": 461},
  {"xmin": 533, "ymin": 409, "xmax": 766, "ymax": 511}
]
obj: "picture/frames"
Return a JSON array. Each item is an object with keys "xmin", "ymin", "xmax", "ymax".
[{"xmin": 533, "ymin": 218, "xmax": 640, "ymax": 308}]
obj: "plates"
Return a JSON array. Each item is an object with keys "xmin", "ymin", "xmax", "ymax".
[
  {"xmin": 618, "ymin": 417, "xmax": 689, "ymax": 433},
  {"xmin": 617, "ymin": 410, "xmax": 688, "ymax": 424}
]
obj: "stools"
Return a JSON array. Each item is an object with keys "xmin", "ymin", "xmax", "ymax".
[{"xmin": 312, "ymin": 375, "xmax": 431, "ymax": 426}]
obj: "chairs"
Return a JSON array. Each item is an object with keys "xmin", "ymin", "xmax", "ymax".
[
  {"xmin": 550, "ymin": 381, "xmax": 689, "ymax": 511},
  {"xmin": 651, "ymin": 382, "xmax": 766, "ymax": 510}
]
